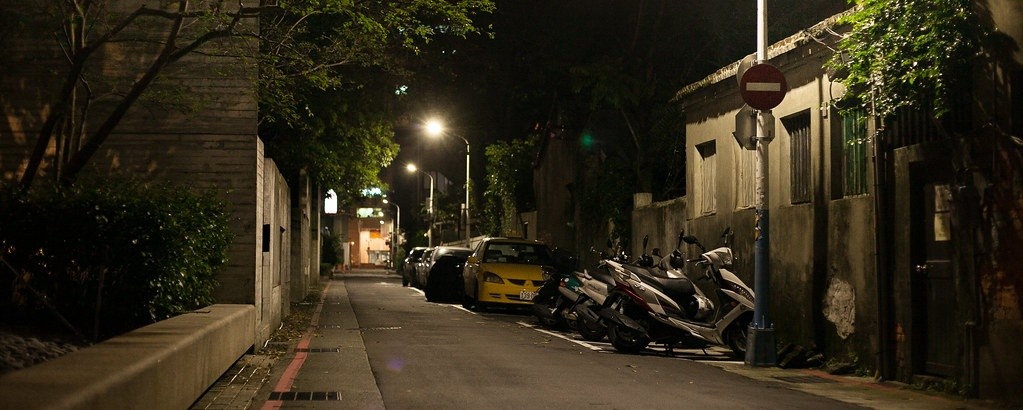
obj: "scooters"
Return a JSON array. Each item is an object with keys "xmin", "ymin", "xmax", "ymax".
[{"xmin": 532, "ymin": 224, "xmax": 754, "ymax": 356}]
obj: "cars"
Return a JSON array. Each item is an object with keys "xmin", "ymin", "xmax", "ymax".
[
  {"xmin": 402, "ymin": 246, "xmax": 475, "ymax": 302},
  {"xmin": 464, "ymin": 237, "xmax": 557, "ymax": 312}
]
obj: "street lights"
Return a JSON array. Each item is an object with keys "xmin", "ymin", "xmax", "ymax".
[
  {"xmin": 406, "ymin": 163, "xmax": 433, "ymax": 248},
  {"xmin": 371, "ymin": 188, "xmax": 400, "ymax": 252},
  {"xmin": 425, "ymin": 120, "xmax": 471, "ymax": 249}
]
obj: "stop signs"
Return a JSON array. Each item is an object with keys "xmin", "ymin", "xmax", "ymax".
[{"xmin": 738, "ymin": 64, "xmax": 788, "ymax": 112}]
